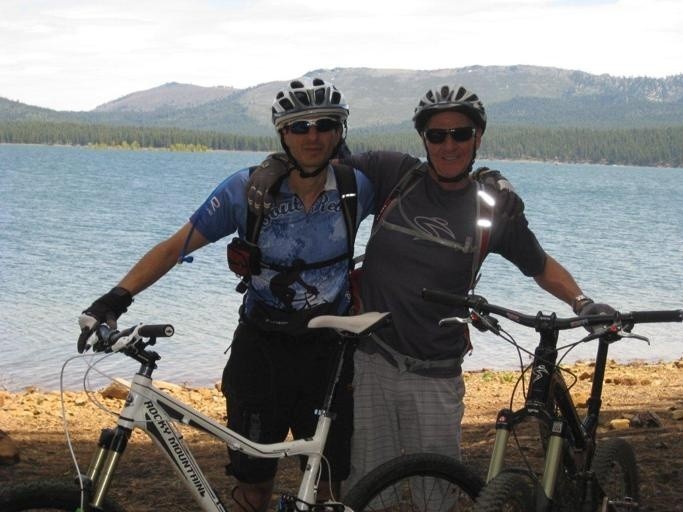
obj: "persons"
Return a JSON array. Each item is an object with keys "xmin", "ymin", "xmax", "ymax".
[
  {"xmin": 330, "ymin": 85, "xmax": 616, "ymax": 511},
  {"xmin": 77, "ymin": 76, "xmax": 379, "ymax": 511}
]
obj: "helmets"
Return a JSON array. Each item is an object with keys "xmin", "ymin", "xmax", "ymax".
[
  {"xmin": 272, "ymin": 78, "xmax": 349, "ymax": 132},
  {"xmin": 412, "ymin": 85, "xmax": 486, "ymax": 135}
]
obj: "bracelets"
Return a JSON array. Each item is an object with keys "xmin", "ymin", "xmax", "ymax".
[{"xmin": 573, "ymin": 294, "xmax": 592, "ymax": 313}]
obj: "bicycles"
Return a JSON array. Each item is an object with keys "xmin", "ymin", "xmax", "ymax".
[
  {"xmin": 336, "ymin": 286, "xmax": 683, "ymax": 511},
  {"xmin": 2, "ymin": 306, "xmax": 492, "ymax": 512}
]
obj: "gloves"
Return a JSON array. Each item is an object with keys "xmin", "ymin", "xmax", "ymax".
[
  {"xmin": 576, "ymin": 300, "xmax": 617, "ymax": 331},
  {"xmin": 246, "ymin": 153, "xmax": 292, "ymax": 216},
  {"xmin": 78, "ymin": 287, "xmax": 132, "ymax": 353},
  {"xmin": 472, "ymin": 166, "xmax": 523, "ymax": 218}
]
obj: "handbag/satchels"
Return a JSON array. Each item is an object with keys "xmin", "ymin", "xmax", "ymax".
[{"xmin": 246, "ymin": 285, "xmax": 334, "ymax": 333}]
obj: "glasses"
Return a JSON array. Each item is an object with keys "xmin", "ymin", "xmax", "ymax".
[
  {"xmin": 424, "ymin": 127, "xmax": 476, "ymax": 144},
  {"xmin": 286, "ymin": 116, "xmax": 337, "ymax": 134}
]
